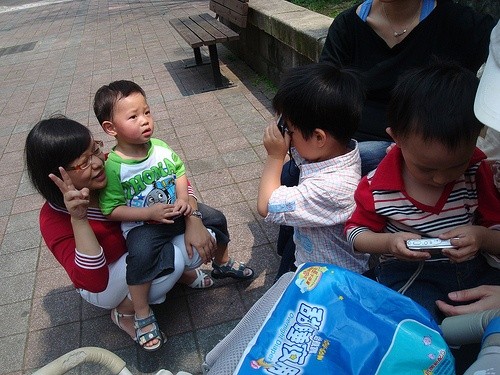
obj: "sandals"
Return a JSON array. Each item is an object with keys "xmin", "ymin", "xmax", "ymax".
[
  {"xmin": 111, "ymin": 307, "xmax": 167, "ymax": 347},
  {"xmin": 133, "ymin": 307, "xmax": 163, "ymax": 352},
  {"xmin": 177, "ymin": 267, "xmax": 214, "ymax": 288},
  {"xmin": 211, "ymin": 261, "xmax": 254, "ymax": 281}
]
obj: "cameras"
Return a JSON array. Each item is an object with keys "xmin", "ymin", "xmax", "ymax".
[
  {"xmin": 277, "ymin": 112, "xmax": 288, "ymax": 138},
  {"xmin": 406, "ymin": 237, "xmax": 460, "ymax": 255}
]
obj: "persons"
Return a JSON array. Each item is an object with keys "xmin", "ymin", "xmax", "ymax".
[
  {"xmin": 23, "ymin": 114, "xmax": 215, "ymax": 346},
  {"xmin": 255, "ymin": 0, "xmax": 500, "ymax": 375},
  {"xmin": 92, "ymin": 80, "xmax": 253, "ymax": 352}
]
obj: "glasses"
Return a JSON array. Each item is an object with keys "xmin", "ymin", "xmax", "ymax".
[{"xmin": 65, "ymin": 140, "xmax": 103, "ymax": 171}]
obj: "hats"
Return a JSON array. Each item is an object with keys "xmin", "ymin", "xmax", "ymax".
[{"xmin": 474, "ymin": 19, "xmax": 500, "ymax": 131}]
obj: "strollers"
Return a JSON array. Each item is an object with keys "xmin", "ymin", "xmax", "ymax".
[{"xmin": 30, "ymin": 262, "xmax": 500, "ymax": 375}]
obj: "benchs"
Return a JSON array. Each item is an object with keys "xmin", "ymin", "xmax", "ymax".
[{"xmin": 169, "ymin": 0, "xmax": 249, "ymax": 93}]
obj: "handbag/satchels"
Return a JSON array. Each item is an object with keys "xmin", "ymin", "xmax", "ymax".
[{"xmin": 234, "ymin": 261, "xmax": 457, "ymax": 375}]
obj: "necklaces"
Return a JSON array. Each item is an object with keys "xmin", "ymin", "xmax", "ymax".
[{"xmin": 381, "ymin": 1, "xmax": 418, "ymax": 42}]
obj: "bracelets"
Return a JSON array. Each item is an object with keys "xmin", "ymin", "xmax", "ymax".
[{"xmin": 187, "ymin": 210, "xmax": 204, "ymax": 217}]
